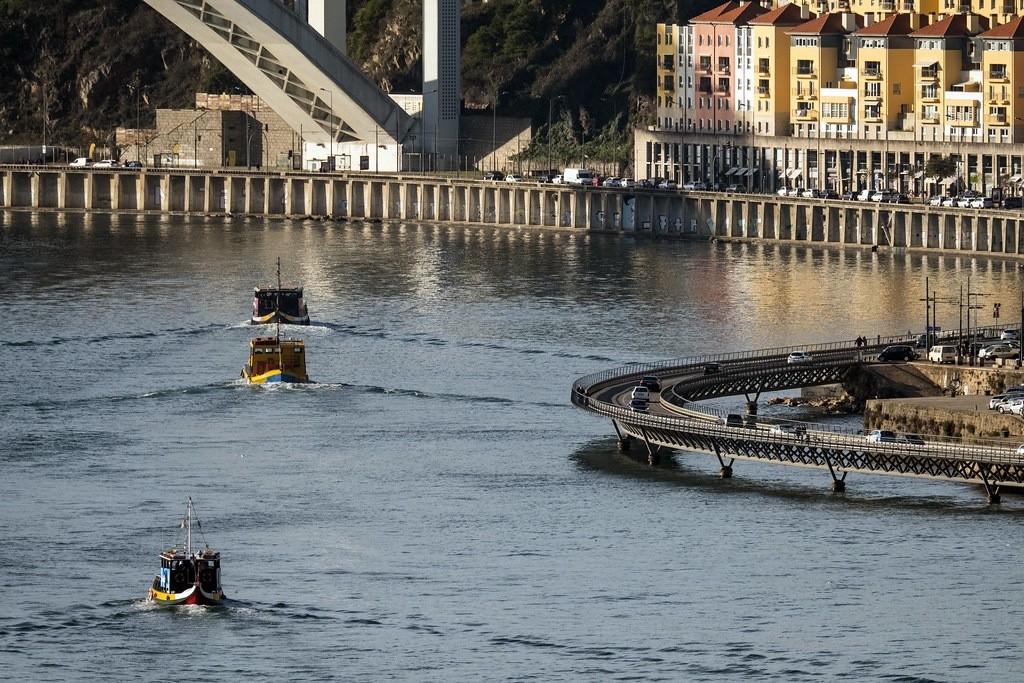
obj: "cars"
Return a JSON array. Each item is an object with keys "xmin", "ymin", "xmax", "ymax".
[
  {"xmin": 631, "ymin": 351, "xmax": 925, "ymax": 445},
  {"xmin": 1015, "ymin": 444, "xmax": 1024, "ymax": 458},
  {"xmin": 506, "ymin": 174, "xmax": 523, "ymax": 182},
  {"xmin": 877, "ymin": 329, "xmax": 1021, "ymax": 364},
  {"xmin": 121, "ymin": 161, "xmax": 142, "ymax": 167},
  {"xmin": 842, "ymin": 188, "xmax": 910, "ymax": 204},
  {"xmin": 539, "ymin": 168, "xmax": 593, "ymax": 185},
  {"xmin": 592, "ymin": 177, "xmax": 635, "ymax": 188},
  {"xmin": 683, "ymin": 180, "xmax": 746, "ymax": 193},
  {"xmin": 777, "ymin": 186, "xmax": 838, "ymax": 199},
  {"xmin": 990, "ymin": 386, "xmax": 1024, "ymax": 420},
  {"xmin": 93, "ymin": 160, "xmax": 119, "ymax": 167},
  {"xmin": 926, "ymin": 196, "xmax": 1023, "ymax": 210},
  {"xmin": 634, "ymin": 177, "xmax": 677, "ymax": 188}
]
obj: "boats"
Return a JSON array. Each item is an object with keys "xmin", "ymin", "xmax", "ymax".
[
  {"xmin": 148, "ymin": 496, "xmax": 224, "ymax": 607},
  {"xmin": 250, "ymin": 257, "xmax": 310, "ymax": 325},
  {"xmin": 240, "ymin": 309, "xmax": 307, "ymax": 384}
]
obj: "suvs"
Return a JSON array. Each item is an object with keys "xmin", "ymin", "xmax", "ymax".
[{"xmin": 483, "ymin": 171, "xmax": 504, "ymax": 180}]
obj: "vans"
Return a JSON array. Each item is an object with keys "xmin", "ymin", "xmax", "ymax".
[{"xmin": 70, "ymin": 158, "xmax": 95, "ymax": 166}]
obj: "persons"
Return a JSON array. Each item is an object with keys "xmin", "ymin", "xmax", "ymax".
[
  {"xmin": 125, "ymin": 160, "xmax": 129, "ymax": 167},
  {"xmin": 796, "ymin": 425, "xmax": 801, "ymax": 436},
  {"xmin": 802, "ymin": 425, "xmax": 806, "ymax": 434},
  {"xmin": 855, "ymin": 335, "xmax": 864, "ymax": 346}
]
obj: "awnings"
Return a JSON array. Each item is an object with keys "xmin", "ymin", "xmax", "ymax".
[
  {"xmin": 725, "ymin": 167, "xmax": 738, "ymax": 175},
  {"xmin": 788, "ymin": 170, "xmax": 802, "ymax": 178},
  {"xmin": 744, "ymin": 168, "xmax": 758, "ymax": 175},
  {"xmin": 924, "ymin": 173, "xmax": 940, "ymax": 183},
  {"xmin": 938, "ymin": 174, "xmax": 961, "ymax": 185},
  {"xmin": 779, "ymin": 169, "xmax": 791, "ymax": 177},
  {"xmin": 911, "ymin": 60, "xmax": 938, "ymax": 67},
  {"xmin": 913, "ymin": 170, "xmax": 923, "ymax": 178},
  {"xmin": 734, "ymin": 167, "xmax": 747, "ymax": 176}
]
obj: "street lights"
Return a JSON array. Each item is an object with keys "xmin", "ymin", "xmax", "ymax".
[{"xmin": 233, "ymin": 85, "xmax": 616, "ymax": 179}]
